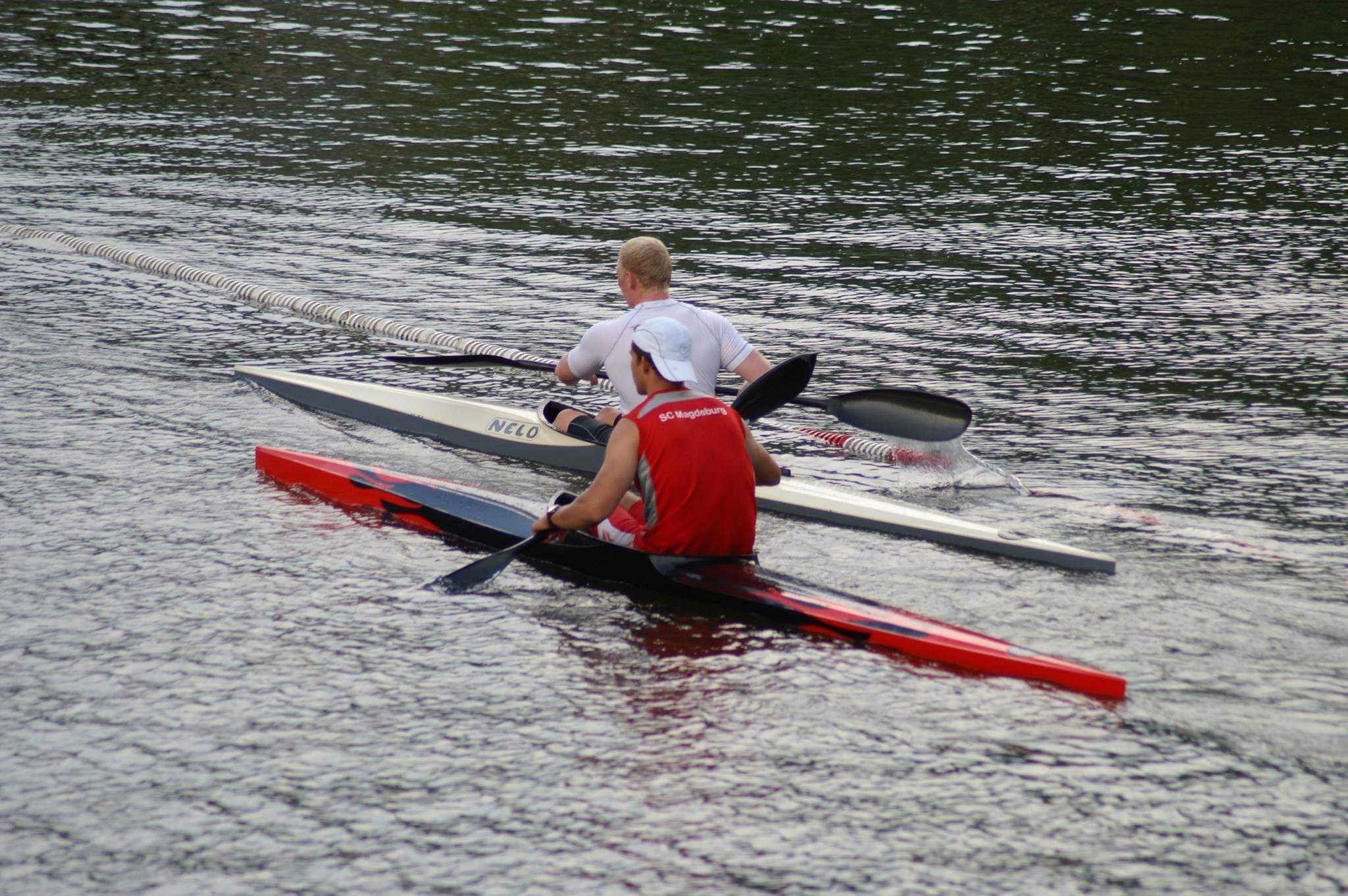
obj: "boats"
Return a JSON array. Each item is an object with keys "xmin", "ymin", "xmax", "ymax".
[
  {"xmin": 254, "ymin": 446, "xmax": 1127, "ymax": 700},
  {"xmin": 234, "ymin": 363, "xmax": 1116, "ymax": 574}
]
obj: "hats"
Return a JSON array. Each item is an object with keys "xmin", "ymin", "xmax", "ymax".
[{"xmin": 632, "ymin": 316, "xmax": 699, "ymax": 385}]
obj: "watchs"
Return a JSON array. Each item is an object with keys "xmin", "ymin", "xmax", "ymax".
[{"xmin": 546, "ymin": 503, "xmax": 560, "ymax": 528}]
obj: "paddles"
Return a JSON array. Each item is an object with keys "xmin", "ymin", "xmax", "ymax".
[
  {"xmin": 386, "ymin": 353, "xmax": 972, "ymax": 441},
  {"xmin": 428, "ymin": 353, "xmax": 817, "ymax": 591}
]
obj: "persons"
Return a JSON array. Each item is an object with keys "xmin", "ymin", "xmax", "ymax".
[
  {"xmin": 531, "ymin": 316, "xmax": 781, "ymax": 559},
  {"xmin": 552, "ymin": 236, "xmax": 772, "ymax": 445}
]
